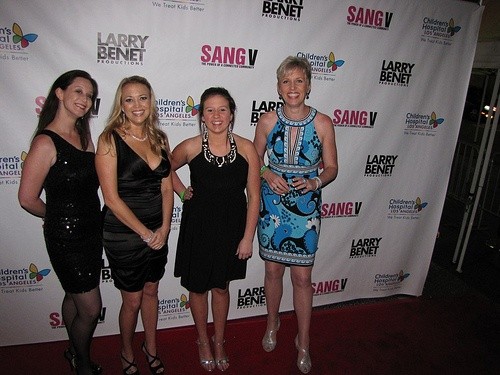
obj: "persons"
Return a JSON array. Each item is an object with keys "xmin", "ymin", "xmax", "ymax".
[
  {"xmin": 169, "ymin": 87, "xmax": 261, "ymax": 373},
  {"xmin": 254, "ymin": 57, "xmax": 338, "ymax": 374},
  {"xmin": 95, "ymin": 76, "xmax": 174, "ymax": 375},
  {"xmin": 18, "ymin": 70, "xmax": 104, "ymax": 375}
]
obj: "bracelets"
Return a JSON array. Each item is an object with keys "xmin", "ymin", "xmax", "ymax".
[
  {"xmin": 310, "ymin": 176, "xmax": 322, "ymax": 192},
  {"xmin": 259, "ymin": 164, "xmax": 268, "ymax": 178},
  {"xmin": 141, "ymin": 230, "xmax": 153, "ymax": 243},
  {"xmin": 180, "ymin": 191, "xmax": 185, "ymax": 203}
]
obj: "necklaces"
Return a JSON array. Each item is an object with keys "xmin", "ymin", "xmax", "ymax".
[
  {"xmin": 125, "ymin": 130, "xmax": 148, "ymax": 142},
  {"xmin": 202, "ymin": 131, "xmax": 237, "ymax": 167}
]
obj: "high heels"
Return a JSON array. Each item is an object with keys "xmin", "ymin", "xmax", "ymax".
[
  {"xmin": 211, "ymin": 334, "xmax": 230, "ymax": 371},
  {"xmin": 294, "ymin": 333, "xmax": 312, "ymax": 374},
  {"xmin": 141, "ymin": 341, "xmax": 165, "ymax": 375},
  {"xmin": 63, "ymin": 348, "xmax": 102, "ymax": 375},
  {"xmin": 262, "ymin": 315, "xmax": 281, "ymax": 352},
  {"xmin": 196, "ymin": 337, "xmax": 215, "ymax": 371},
  {"xmin": 119, "ymin": 350, "xmax": 140, "ymax": 375}
]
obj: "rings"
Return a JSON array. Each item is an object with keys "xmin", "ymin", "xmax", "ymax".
[{"xmin": 302, "ymin": 178, "xmax": 307, "ymax": 184}]
